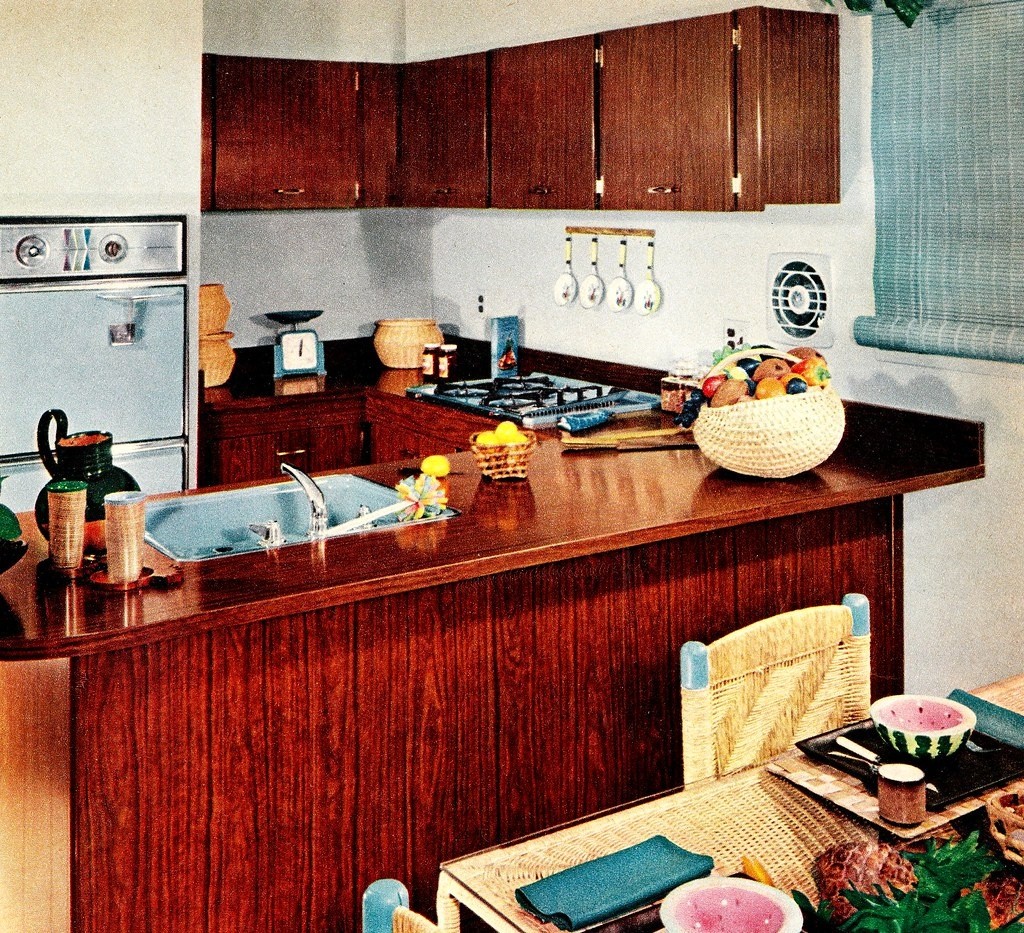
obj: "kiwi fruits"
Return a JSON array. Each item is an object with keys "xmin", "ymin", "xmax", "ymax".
[{"xmin": 710, "ymin": 347, "xmax": 826, "ymax": 407}]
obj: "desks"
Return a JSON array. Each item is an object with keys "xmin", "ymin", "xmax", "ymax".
[{"xmin": 435, "ymin": 674, "xmax": 1024, "ymax": 933}]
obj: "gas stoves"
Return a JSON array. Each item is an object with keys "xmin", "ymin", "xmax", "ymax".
[{"xmin": 405, "ymin": 369, "xmax": 662, "ymax": 425}]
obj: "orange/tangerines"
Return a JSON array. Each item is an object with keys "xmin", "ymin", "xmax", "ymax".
[
  {"xmin": 780, "ymin": 373, "xmax": 809, "ymax": 390},
  {"xmin": 756, "ymin": 377, "xmax": 787, "ymax": 400}
]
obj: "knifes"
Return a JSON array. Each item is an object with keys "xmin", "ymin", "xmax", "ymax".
[{"xmin": 836, "ymin": 736, "xmax": 939, "ymax": 794}]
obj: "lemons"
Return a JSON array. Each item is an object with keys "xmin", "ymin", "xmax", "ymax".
[
  {"xmin": 475, "ymin": 421, "xmax": 528, "ymax": 446},
  {"xmin": 420, "ymin": 455, "xmax": 452, "ymax": 478}
]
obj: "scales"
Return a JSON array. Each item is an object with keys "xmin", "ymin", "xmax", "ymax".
[{"xmin": 264, "ymin": 309, "xmax": 327, "ymax": 377}]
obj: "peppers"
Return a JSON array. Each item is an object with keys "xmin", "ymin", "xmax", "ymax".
[{"xmin": 790, "ymin": 356, "xmax": 831, "ymax": 388}]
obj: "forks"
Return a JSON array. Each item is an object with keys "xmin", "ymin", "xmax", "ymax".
[{"xmin": 965, "ymin": 740, "xmax": 1001, "ymax": 752}]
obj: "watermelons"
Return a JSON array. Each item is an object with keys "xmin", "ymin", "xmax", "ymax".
[
  {"xmin": 867, "ymin": 694, "xmax": 977, "ymax": 760},
  {"xmin": 659, "ymin": 876, "xmax": 804, "ymax": 933}
]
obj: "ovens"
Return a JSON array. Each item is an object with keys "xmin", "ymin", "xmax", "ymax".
[{"xmin": 0, "ymin": 214, "xmax": 193, "ymax": 511}]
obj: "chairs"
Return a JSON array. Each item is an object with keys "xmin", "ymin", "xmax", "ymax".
[{"xmin": 679, "ymin": 593, "xmax": 873, "ymax": 784}]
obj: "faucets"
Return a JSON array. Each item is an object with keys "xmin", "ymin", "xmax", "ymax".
[{"xmin": 279, "ymin": 462, "xmax": 330, "ymax": 536}]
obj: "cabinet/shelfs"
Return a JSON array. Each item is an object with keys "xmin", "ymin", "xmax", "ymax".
[
  {"xmin": 200, "ymin": 5, "xmax": 841, "ymax": 214},
  {"xmin": 197, "ymin": 408, "xmax": 522, "ymax": 484}
]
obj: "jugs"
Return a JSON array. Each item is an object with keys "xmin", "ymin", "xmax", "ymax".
[{"xmin": 33, "ymin": 409, "xmax": 141, "ymax": 540}]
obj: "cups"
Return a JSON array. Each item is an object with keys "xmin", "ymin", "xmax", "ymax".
[
  {"xmin": 877, "ymin": 763, "xmax": 927, "ymax": 827},
  {"xmin": 46, "ymin": 480, "xmax": 88, "ymax": 569},
  {"xmin": 104, "ymin": 491, "xmax": 146, "ymax": 583}
]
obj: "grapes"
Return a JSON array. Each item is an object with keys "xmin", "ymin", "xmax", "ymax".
[{"xmin": 712, "ymin": 343, "xmax": 750, "ymax": 365}]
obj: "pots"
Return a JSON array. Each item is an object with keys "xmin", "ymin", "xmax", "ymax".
[
  {"xmin": 554, "ymin": 237, "xmax": 577, "ymax": 306},
  {"xmin": 606, "ymin": 241, "xmax": 634, "ymax": 312},
  {"xmin": 635, "ymin": 242, "xmax": 662, "ymax": 314},
  {"xmin": 580, "ymin": 238, "xmax": 605, "ymax": 309}
]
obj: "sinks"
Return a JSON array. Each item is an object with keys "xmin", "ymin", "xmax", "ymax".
[{"xmin": 143, "ymin": 477, "xmax": 432, "ymax": 549}]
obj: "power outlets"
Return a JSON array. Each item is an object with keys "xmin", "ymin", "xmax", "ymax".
[{"xmin": 724, "ymin": 318, "xmax": 751, "ymax": 351}]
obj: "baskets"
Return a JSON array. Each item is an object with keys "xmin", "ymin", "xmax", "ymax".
[
  {"xmin": 374, "ymin": 318, "xmax": 443, "ymax": 368},
  {"xmin": 469, "ymin": 429, "xmax": 536, "ymax": 479},
  {"xmin": 693, "ymin": 348, "xmax": 844, "ymax": 478},
  {"xmin": 198, "ymin": 284, "xmax": 230, "ymax": 335},
  {"xmin": 198, "ymin": 331, "xmax": 236, "ymax": 389}
]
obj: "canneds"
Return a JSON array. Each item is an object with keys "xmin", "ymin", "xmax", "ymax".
[{"xmin": 421, "ymin": 343, "xmax": 457, "ymax": 382}]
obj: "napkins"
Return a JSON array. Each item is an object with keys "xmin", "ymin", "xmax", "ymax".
[
  {"xmin": 514, "ymin": 834, "xmax": 715, "ymax": 929},
  {"xmin": 944, "ymin": 688, "xmax": 1023, "ymax": 747}
]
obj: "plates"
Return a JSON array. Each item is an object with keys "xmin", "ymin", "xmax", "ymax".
[{"xmin": 794, "ymin": 719, "xmax": 1024, "ymax": 810}]
